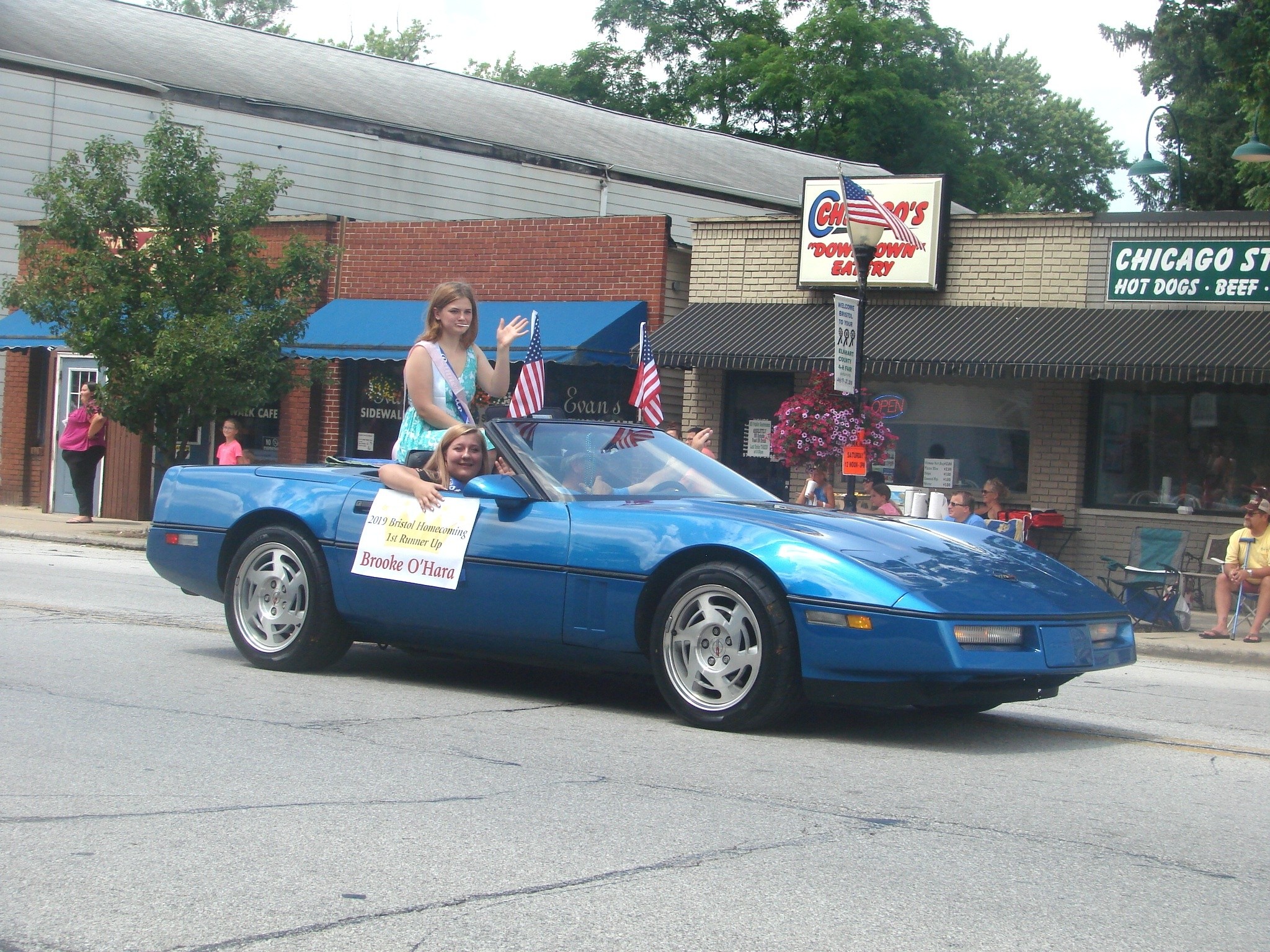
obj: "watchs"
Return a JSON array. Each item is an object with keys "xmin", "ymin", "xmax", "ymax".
[{"xmin": 1247, "ymin": 569, "xmax": 1252, "ymax": 577}]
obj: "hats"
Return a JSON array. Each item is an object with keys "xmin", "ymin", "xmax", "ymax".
[
  {"xmin": 561, "ymin": 429, "xmax": 611, "ymax": 458},
  {"xmin": 1245, "ymin": 498, "xmax": 1270, "ymax": 515}
]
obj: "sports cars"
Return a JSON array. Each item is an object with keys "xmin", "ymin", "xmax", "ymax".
[{"xmin": 147, "ymin": 417, "xmax": 1137, "ymax": 733}]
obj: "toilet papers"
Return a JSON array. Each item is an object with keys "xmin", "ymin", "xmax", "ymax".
[
  {"xmin": 1160, "ymin": 476, "xmax": 1173, "ymax": 503},
  {"xmin": 927, "ymin": 491, "xmax": 949, "ymax": 521},
  {"xmin": 910, "ymin": 492, "xmax": 927, "ymax": 519}
]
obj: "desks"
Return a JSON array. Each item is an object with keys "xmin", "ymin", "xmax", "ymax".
[{"xmin": 1021, "ymin": 527, "xmax": 1082, "ymax": 561}]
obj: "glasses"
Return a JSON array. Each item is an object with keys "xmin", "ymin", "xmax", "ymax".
[
  {"xmin": 1244, "ymin": 510, "xmax": 1261, "ymax": 517},
  {"xmin": 982, "ymin": 490, "xmax": 992, "ymax": 493},
  {"xmin": 948, "ymin": 500, "xmax": 963, "ymax": 506},
  {"xmin": 863, "ymin": 478, "xmax": 874, "ymax": 483}
]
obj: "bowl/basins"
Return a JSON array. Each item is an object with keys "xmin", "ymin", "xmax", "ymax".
[{"xmin": 1176, "ymin": 506, "xmax": 1194, "ymax": 515}]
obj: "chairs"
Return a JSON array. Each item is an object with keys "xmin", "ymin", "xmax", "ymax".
[
  {"xmin": 1096, "ymin": 526, "xmax": 1191, "ymax": 630},
  {"xmin": 1179, "ymin": 531, "xmax": 1233, "ymax": 617},
  {"xmin": 1226, "ymin": 590, "xmax": 1270, "ymax": 636}
]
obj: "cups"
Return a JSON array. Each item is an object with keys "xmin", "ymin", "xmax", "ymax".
[{"xmin": 804, "ymin": 480, "xmax": 818, "ymax": 498}]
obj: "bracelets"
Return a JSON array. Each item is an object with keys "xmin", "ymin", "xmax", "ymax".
[{"xmin": 823, "ymin": 502, "xmax": 825, "ymax": 507}]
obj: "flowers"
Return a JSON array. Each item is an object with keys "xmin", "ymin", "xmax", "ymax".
[{"xmin": 763, "ymin": 375, "xmax": 897, "ymax": 474}]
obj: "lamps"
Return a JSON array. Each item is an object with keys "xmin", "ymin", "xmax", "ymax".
[
  {"xmin": 1230, "ymin": 98, "xmax": 1270, "ymax": 163},
  {"xmin": 1126, "ymin": 106, "xmax": 1184, "ymax": 210}
]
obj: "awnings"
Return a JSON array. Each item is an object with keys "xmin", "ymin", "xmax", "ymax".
[
  {"xmin": 629, "ymin": 302, "xmax": 1270, "ymax": 391},
  {"xmin": 269, "ymin": 299, "xmax": 648, "ymax": 370},
  {"xmin": 0, "ymin": 298, "xmax": 288, "ymax": 349}
]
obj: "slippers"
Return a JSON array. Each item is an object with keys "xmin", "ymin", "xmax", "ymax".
[
  {"xmin": 1198, "ymin": 628, "xmax": 1230, "ymax": 639},
  {"xmin": 1243, "ymin": 633, "xmax": 1261, "ymax": 643},
  {"xmin": 66, "ymin": 518, "xmax": 91, "ymax": 523}
]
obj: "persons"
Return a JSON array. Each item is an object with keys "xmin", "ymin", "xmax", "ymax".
[
  {"xmin": 397, "ymin": 282, "xmax": 529, "ymax": 474},
  {"xmin": 1199, "ymin": 498, "xmax": 1270, "ymax": 643},
  {"xmin": 796, "ymin": 465, "xmax": 835, "ymax": 509},
  {"xmin": 378, "ymin": 424, "xmax": 516, "ymax": 513},
  {"xmin": 58, "ymin": 381, "xmax": 109, "ymax": 523},
  {"xmin": 856, "ymin": 471, "xmax": 904, "ymax": 516},
  {"xmin": 560, "ymin": 428, "xmax": 717, "ymax": 494},
  {"xmin": 666, "ymin": 427, "xmax": 715, "ymax": 496},
  {"xmin": 215, "ymin": 418, "xmax": 244, "ymax": 465},
  {"xmin": 973, "ymin": 477, "xmax": 1007, "ymax": 520},
  {"xmin": 943, "ymin": 491, "xmax": 987, "ymax": 530}
]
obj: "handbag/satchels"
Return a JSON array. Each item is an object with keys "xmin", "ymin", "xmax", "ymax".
[{"xmin": 997, "ymin": 509, "xmax": 1064, "ymax": 528}]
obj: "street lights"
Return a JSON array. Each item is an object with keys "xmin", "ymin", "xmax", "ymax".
[{"xmin": 843, "ymin": 188, "xmax": 885, "ymax": 515}]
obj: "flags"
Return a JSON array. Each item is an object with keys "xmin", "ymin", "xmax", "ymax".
[
  {"xmin": 843, "ymin": 174, "xmax": 926, "ymax": 252},
  {"xmin": 600, "ymin": 427, "xmax": 655, "ymax": 454},
  {"xmin": 628, "ymin": 322, "xmax": 664, "ymax": 428},
  {"xmin": 506, "ymin": 310, "xmax": 545, "ymax": 441},
  {"xmin": 834, "ymin": 299, "xmax": 859, "ymax": 395}
]
